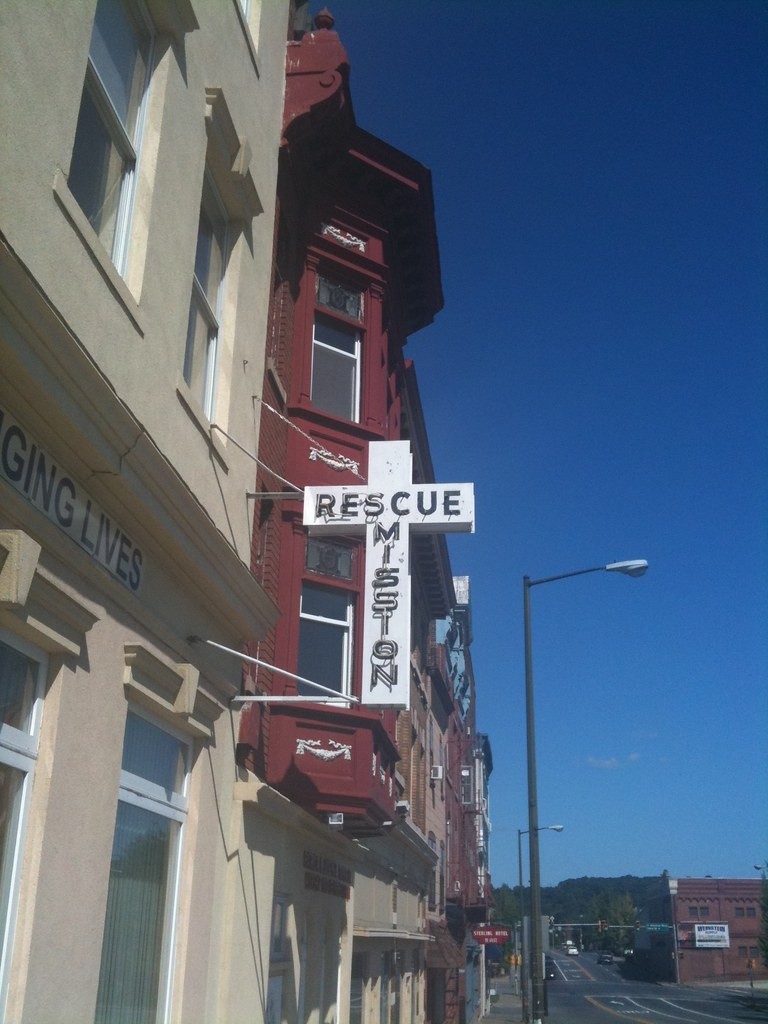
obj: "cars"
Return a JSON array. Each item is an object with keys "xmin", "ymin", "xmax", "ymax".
[{"xmin": 567, "ymin": 946, "xmax": 579, "ymax": 956}]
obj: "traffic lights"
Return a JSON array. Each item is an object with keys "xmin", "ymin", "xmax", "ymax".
[
  {"xmin": 603, "ymin": 923, "xmax": 608, "ymax": 933},
  {"xmin": 635, "ymin": 920, "xmax": 641, "ymax": 935},
  {"xmin": 678, "ymin": 951, "xmax": 684, "ymax": 960}
]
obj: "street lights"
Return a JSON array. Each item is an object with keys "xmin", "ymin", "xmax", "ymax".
[
  {"xmin": 518, "ymin": 825, "xmax": 564, "ymax": 993},
  {"xmin": 522, "ymin": 557, "xmax": 649, "ymax": 1024}
]
